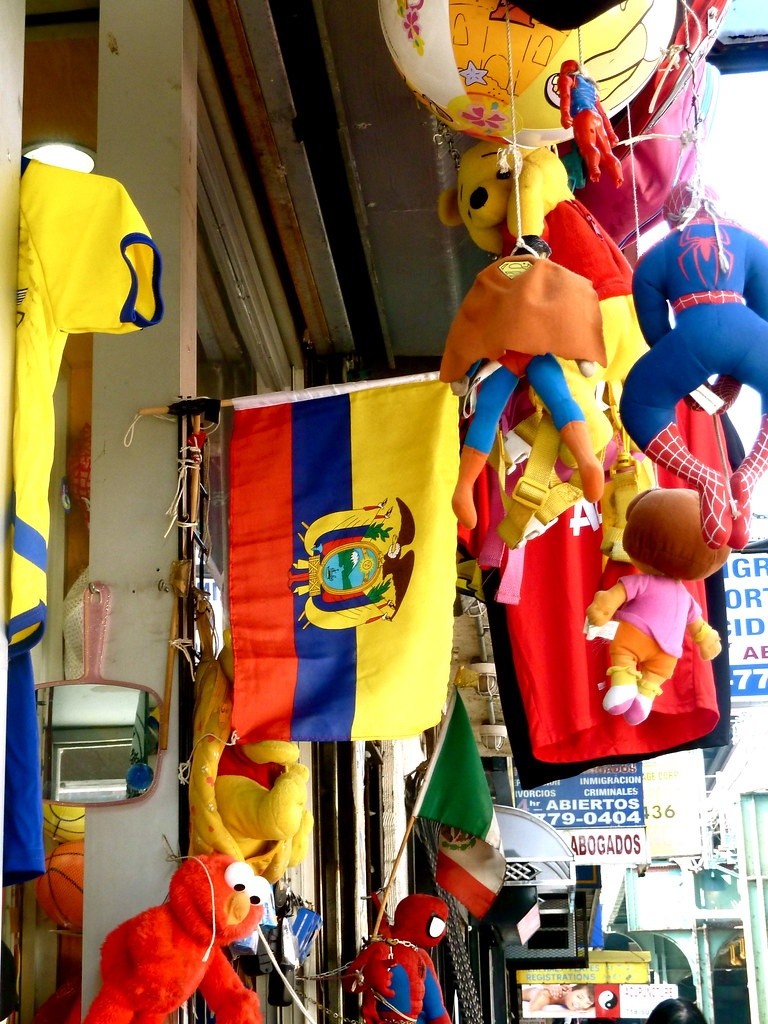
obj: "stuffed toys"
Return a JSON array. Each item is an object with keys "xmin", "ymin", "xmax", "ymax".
[
  {"xmin": 438, "ymin": 142, "xmax": 656, "ymax": 562},
  {"xmin": 585, "ymin": 487, "xmax": 732, "ymax": 724},
  {"xmin": 187, "ymin": 627, "xmax": 314, "ymax": 885},
  {"xmin": 83, "ymin": 852, "xmax": 272, "ymax": 1024}
]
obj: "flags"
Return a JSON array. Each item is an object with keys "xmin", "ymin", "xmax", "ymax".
[
  {"xmin": 411, "ymin": 685, "xmax": 507, "ymax": 919},
  {"xmin": 230, "ymin": 372, "xmax": 460, "ymax": 744}
]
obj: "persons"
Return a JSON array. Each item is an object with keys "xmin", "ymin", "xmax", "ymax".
[
  {"xmin": 645, "ymin": 998, "xmax": 707, "ymax": 1024},
  {"xmin": 619, "ymin": 181, "xmax": 768, "ymax": 549},
  {"xmin": 440, "ymin": 234, "xmax": 608, "ymax": 529},
  {"xmin": 343, "ymin": 894, "xmax": 453, "ymax": 1024},
  {"xmin": 522, "ymin": 983, "xmax": 595, "ymax": 1011},
  {"xmin": 557, "ymin": 60, "xmax": 624, "ymax": 189}
]
